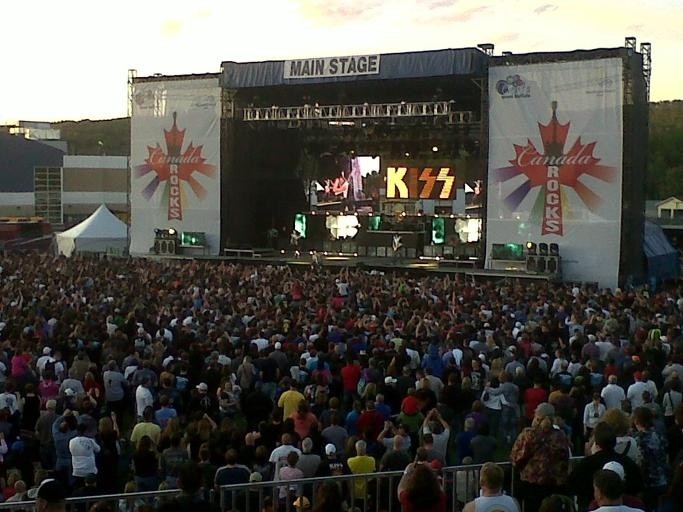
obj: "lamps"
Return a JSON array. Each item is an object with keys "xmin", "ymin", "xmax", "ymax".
[
  {"xmin": 526, "ymin": 241, "xmax": 559, "ymax": 255},
  {"xmin": 153, "ymin": 227, "xmax": 176, "ymax": 239}
]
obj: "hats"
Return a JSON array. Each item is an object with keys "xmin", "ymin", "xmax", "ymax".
[{"xmin": 0, "ymin": 335, "xmax": 670, "ymax": 481}]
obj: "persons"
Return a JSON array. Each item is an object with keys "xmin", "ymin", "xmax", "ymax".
[{"xmin": 305, "ymin": 169, "xmax": 387, "ymax": 207}]
